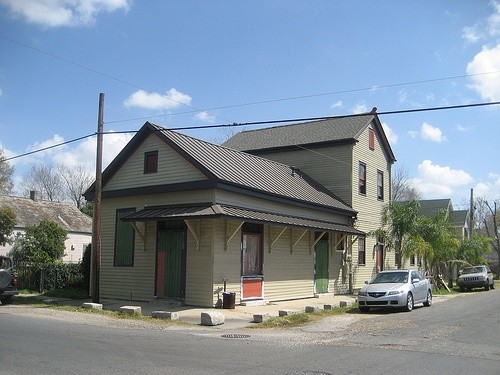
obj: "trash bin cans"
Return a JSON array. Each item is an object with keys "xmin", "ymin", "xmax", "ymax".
[{"xmin": 222, "ymin": 291, "xmax": 236, "ymax": 309}]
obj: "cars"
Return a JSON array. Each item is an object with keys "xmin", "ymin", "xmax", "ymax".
[
  {"xmin": 358, "ymin": 269, "xmax": 434, "ymax": 311},
  {"xmin": 457, "ymin": 266, "xmax": 493, "ymax": 292}
]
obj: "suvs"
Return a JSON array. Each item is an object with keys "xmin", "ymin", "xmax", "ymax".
[{"xmin": 0, "ymin": 255, "xmax": 20, "ymax": 302}]
